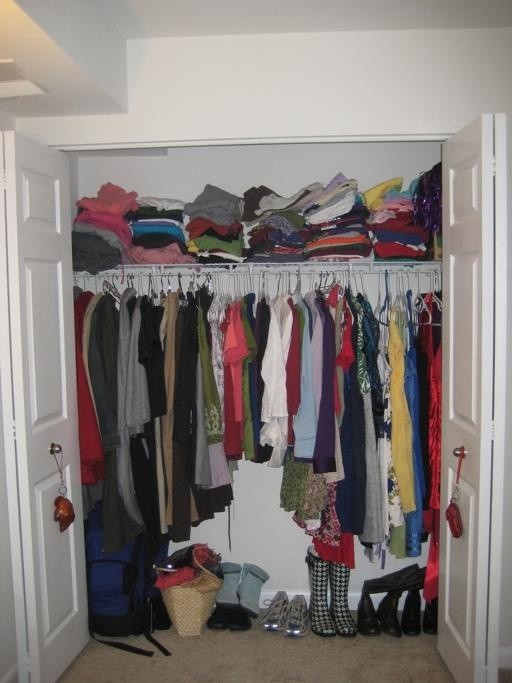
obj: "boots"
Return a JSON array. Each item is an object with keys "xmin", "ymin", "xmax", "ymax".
[
  {"xmin": 238, "ymin": 564, "xmax": 269, "ymax": 615},
  {"xmin": 330, "ymin": 560, "xmax": 357, "ymax": 636},
  {"xmin": 305, "ymin": 546, "xmax": 336, "ymax": 636},
  {"xmin": 216, "ymin": 562, "xmax": 241, "ymax": 606},
  {"xmin": 358, "ymin": 563, "xmax": 438, "ymax": 636},
  {"xmin": 207, "ymin": 603, "xmax": 258, "ymax": 630}
]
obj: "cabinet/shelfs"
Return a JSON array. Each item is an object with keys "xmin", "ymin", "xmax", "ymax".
[{"xmin": 1, "ymin": 111, "xmax": 507, "ymax": 683}]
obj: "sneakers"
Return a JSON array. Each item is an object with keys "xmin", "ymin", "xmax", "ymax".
[
  {"xmin": 283, "ymin": 595, "xmax": 310, "ymax": 637},
  {"xmin": 262, "ymin": 591, "xmax": 289, "ymax": 631}
]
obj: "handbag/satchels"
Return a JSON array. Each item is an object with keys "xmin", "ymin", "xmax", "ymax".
[
  {"xmin": 159, "ymin": 585, "xmax": 218, "ymax": 637},
  {"xmin": 445, "ymin": 503, "xmax": 463, "ymax": 538}
]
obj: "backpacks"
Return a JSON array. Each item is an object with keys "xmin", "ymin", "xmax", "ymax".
[{"xmin": 84, "ymin": 500, "xmax": 171, "ymax": 636}]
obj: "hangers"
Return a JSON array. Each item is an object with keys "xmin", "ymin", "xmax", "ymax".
[{"xmin": 73, "ymin": 262, "xmax": 444, "ymax": 329}]
